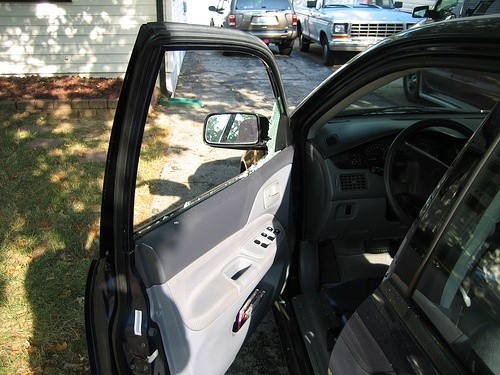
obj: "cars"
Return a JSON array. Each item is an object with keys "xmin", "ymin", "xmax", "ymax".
[
  {"xmin": 407, "ymin": 0, "xmax": 500, "ymax": 32},
  {"xmin": 86, "ymin": 14, "xmax": 500, "ymax": 375}
]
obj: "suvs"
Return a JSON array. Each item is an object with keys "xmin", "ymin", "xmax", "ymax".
[{"xmin": 209, "ymin": 0, "xmax": 297, "ymax": 56}]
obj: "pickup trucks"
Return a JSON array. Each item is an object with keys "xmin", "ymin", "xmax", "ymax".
[{"xmin": 291, "ymin": 0, "xmax": 424, "ymax": 68}]
